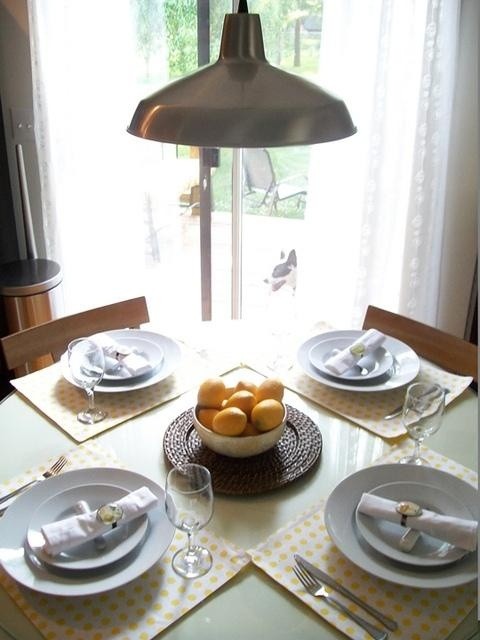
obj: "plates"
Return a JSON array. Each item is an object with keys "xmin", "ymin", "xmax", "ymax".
[
  {"xmin": 27, "ymin": 482, "xmax": 149, "ymax": 570},
  {"xmin": 309, "ymin": 336, "xmax": 395, "ymax": 381},
  {"xmin": 79, "ymin": 335, "xmax": 164, "ymax": 380},
  {"xmin": 297, "ymin": 328, "xmax": 419, "ymax": 393},
  {"xmin": 61, "ymin": 329, "xmax": 181, "ymax": 395},
  {"xmin": 356, "ymin": 481, "xmax": 477, "ymax": 566},
  {"xmin": 0, "ymin": 468, "xmax": 178, "ymax": 599},
  {"xmin": 323, "ymin": 464, "xmax": 478, "ymax": 591}
]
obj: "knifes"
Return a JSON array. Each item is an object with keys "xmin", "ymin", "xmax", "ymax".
[
  {"xmin": 384, "ymin": 384, "xmax": 440, "ymax": 422},
  {"xmin": 295, "ymin": 554, "xmax": 399, "ymax": 632}
]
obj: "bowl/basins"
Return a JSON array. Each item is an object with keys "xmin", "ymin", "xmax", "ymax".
[{"xmin": 193, "ymin": 403, "xmax": 288, "ymax": 459}]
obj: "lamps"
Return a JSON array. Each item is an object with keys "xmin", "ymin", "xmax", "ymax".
[{"xmin": 128, "ymin": 0, "xmax": 356, "ymax": 319}]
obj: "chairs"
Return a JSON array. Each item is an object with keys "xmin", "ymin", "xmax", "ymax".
[
  {"xmin": 243, "ymin": 144, "xmax": 308, "ymax": 218},
  {"xmin": 2, "ymin": 296, "xmax": 151, "ymax": 375},
  {"xmin": 361, "ymin": 305, "xmax": 479, "ymax": 390}
]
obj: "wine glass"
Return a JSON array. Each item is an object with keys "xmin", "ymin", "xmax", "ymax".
[
  {"xmin": 164, "ymin": 464, "xmax": 215, "ymax": 579},
  {"xmin": 69, "ymin": 337, "xmax": 108, "ymax": 428},
  {"xmin": 402, "ymin": 383, "xmax": 446, "ymax": 469}
]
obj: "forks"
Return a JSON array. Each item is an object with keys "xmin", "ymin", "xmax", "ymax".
[
  {"xmin": 0, "ymin": 457, "xmax": 68, "ymax": 508},
  {"xmin": 291, "ymin": 562, "xmax": 387, "ymax": 640}
]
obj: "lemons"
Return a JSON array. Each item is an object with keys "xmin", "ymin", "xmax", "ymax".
[{"xmin": 197, "ymin": 379, "xmax": 284, "ymax": 436}]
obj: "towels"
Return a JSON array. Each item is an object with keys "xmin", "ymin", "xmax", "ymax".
[
  {"xmin": 40, "ymin": 485, "xmax": 161, "ymax": 560},
  {"xmin": 356, "ymin": 492, "xmax": 479, "ymax": 555},
  {"xmin": 88, "ymin": 332, "xmax": 150, "ymax": 373},
  {"xmin": 324, "ymin": 327, "xmax": 386, "ymax": 375}
]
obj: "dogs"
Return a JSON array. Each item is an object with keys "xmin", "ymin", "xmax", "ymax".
[{"xmin": 262, "ymin": 248, "xmax": 297, "ymax": 296}]
